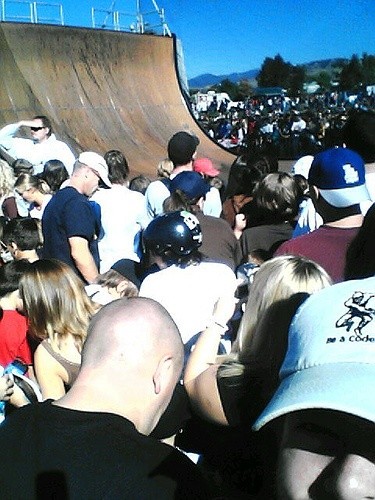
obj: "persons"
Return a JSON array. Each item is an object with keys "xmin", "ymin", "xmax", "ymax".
[
  {"xmin": 193, "ymin": 91, "xmax": 375, "ymax": 159},
  {"xmin": 0, "ymin": 115, "xmax": 375, "ymax": 500}
]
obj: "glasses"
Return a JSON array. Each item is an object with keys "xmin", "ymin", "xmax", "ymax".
[{"xmin": 30, "ymin": 127, "xmax": 45, "ymax": 131}]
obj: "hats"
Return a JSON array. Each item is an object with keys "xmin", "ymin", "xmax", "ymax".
[
  {"xmin": 79, "ymin": 151, "xmax": 113, "ymax": 187},
  {"xmin": 193, "ymin": 158, "xmax": 219, "ymax": 177},
  {"xmin": 171, "ymin": 171, "xmax": 210, "ymax": 199},
  {"xmin": 252, "ymin": 275, "xmax": 375, "ymax": 432},
  {"xmin": 308, "ymin": 147, "xmax": 372, "ymax": 207},
  {"xmin": 111, "ymin": 258, "xmax": 143, "ymax": 290},
  {"xmin": 291, "ymin": 155, "xmax": 314, "ymax": 179},
  {"xmin": 168, "ymin": 131, "xmax": 200, "ymax": 160}
]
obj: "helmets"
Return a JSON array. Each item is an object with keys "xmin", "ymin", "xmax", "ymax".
[{"xmin": 144, "ymin": 211, "xmax": 203, "ymax": 256}]
